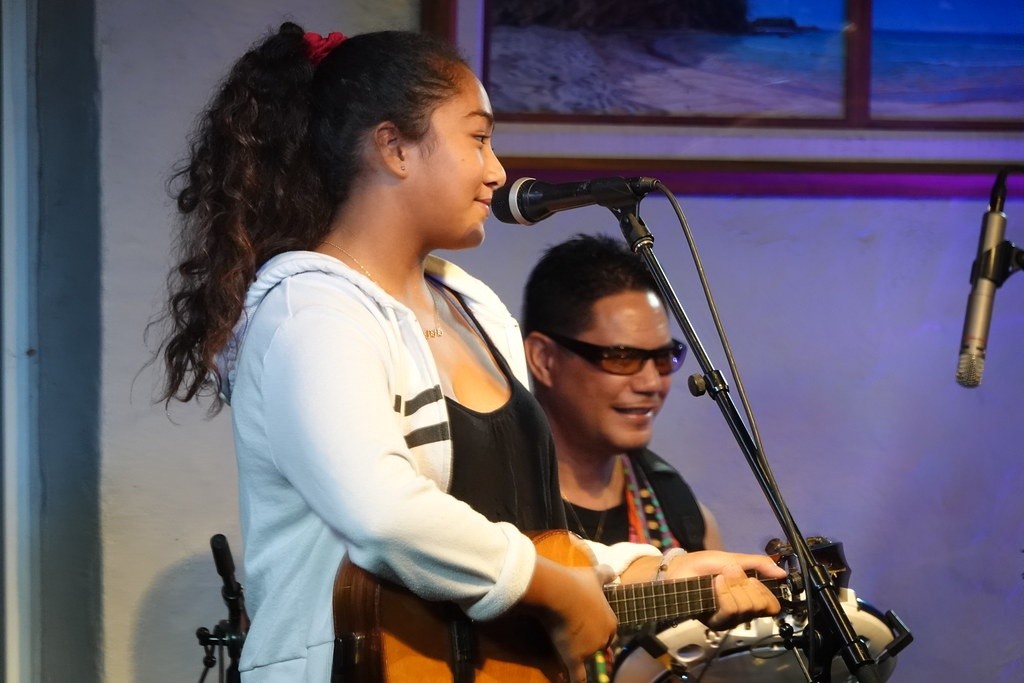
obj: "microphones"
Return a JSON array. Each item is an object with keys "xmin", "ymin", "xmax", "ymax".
[
  {"xmin": 494, "ymin": 172, "xmax": 659, "ymax": 226},
  {"xmin": 954, "ymin": 209, "xmax": 1007, "ymax": 388}
]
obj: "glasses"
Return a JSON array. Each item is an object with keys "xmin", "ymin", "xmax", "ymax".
[{"xmin": 551, "ymin": 331, "xmax": 688, "ymax": 377}]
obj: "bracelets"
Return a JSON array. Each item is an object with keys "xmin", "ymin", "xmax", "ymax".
[{"xmin": 654, "ymin": 546, "xmax": 691, "ymax": 584}]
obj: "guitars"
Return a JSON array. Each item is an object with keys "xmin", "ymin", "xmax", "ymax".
[{"xmin": 329, "ymin": 535, "xmax": 850, "ymax": 683}]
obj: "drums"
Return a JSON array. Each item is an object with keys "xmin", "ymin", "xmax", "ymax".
[{"xmin": 613, "ymin": 596, "xmax": 899, "ymax": 683}]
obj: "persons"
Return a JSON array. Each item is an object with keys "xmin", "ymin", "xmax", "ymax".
[
  {"xmin": 160, "ymin": 24, "xmax": 787, "ymax": 683},
  {"xmin": 524, "ymin": 232, "xmax": 725, "ymax": 554}
]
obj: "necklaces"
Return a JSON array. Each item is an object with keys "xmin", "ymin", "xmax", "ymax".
[{"xmin": 555, "ymin": 457, "xmax": 621, "ymax": 543}]
